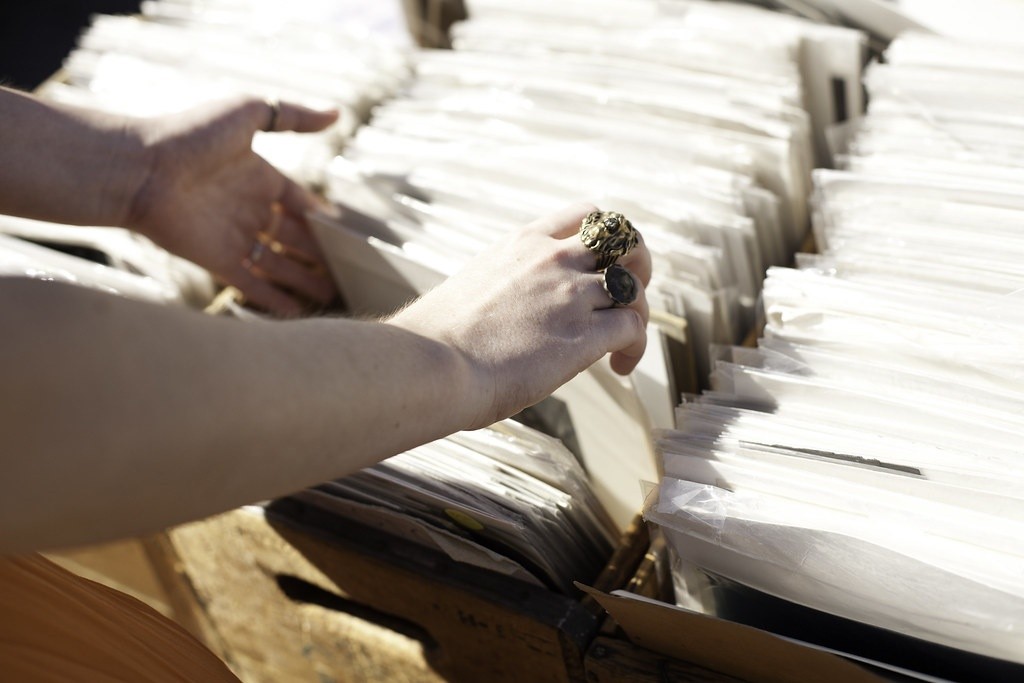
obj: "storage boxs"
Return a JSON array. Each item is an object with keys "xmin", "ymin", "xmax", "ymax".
[{"xmin": 148, "ymin": 231, "xmax": 1024, "ymax": 683}]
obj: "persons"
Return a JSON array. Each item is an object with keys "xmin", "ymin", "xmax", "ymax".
[{"xmin": 0, "ymin": 85, "xmax": 654, "ymax": 554}]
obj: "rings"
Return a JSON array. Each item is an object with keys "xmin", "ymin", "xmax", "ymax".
[
  {"xmin": 263, "ymin": 99, "xmax": 281, "ymax": 132},
  {"xmin": 599, "ymin": 264, "xmax": 638, "ymax": 308},
  {"xmin": 580, "ymin": 211, "xmax": 639, "ymax": 272}
]
